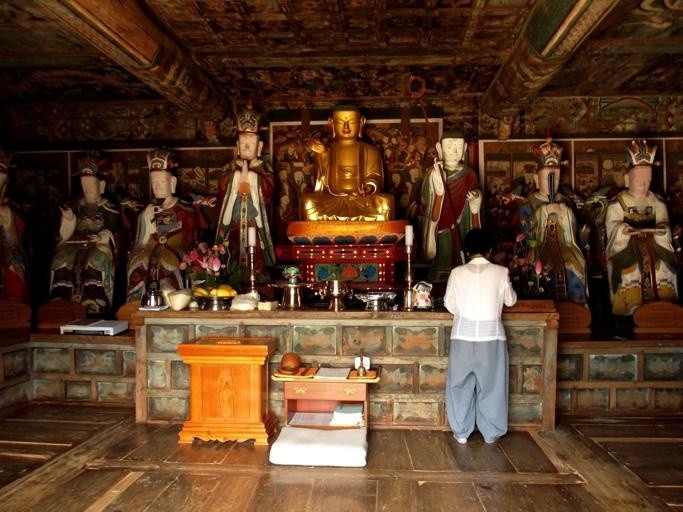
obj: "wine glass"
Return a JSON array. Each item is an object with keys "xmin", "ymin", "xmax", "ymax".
[
  {"xmin": 326, "ymin": 279, "xmax": 349, "ymax": 312},
  {"xmin": 355, "ymin": 292, "xmax": 397, "ymax": 312},
  {"xmin": 199, "ymin": 295, "xmax": 234, "ymax": 312}
]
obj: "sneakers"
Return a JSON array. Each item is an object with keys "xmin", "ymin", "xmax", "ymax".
[
  {"xmin": 484, "ymin": 440, "xmax": 495, "ymax": 443},
  {"xmin": 453, "ymin": 435, "xmax": 467, "ymax": 444}
]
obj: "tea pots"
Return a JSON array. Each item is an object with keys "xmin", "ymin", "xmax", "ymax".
[{"xmin": 141, "ymin": 279, "xmax": 164, "ymax": 309}]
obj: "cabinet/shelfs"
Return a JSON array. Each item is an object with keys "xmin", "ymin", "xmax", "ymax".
[{"xmin": 174, "ymin": 337, "xmax": 379, "ymax": 448}]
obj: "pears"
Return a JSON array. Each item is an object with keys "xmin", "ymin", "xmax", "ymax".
[{"xmin": 210, "ymin": 284, "xmax": 237, "ymax": 297}]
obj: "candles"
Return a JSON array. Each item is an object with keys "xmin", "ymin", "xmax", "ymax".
[
  {"xmin": 332, "ymin": 279, "xmax": 338, "ymax": 294},
  {"xmin": 247, "ymin": 226, "xmax": 257, "ymax": 247},
  {"xmin": 404, "ymin": 225, "xmax": 412, "ymax": 246}
]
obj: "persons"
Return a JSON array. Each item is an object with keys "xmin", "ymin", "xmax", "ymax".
[{"xmin": 443, "ymin": 228, "xmax": 517, "ymax": 444}]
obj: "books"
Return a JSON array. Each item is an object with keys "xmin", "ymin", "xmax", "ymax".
[{"xmin": 329, "ymin": 403, "xmax": 363, "ymax": 426}]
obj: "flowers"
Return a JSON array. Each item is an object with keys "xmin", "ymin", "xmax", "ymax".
[
  {"xmin": 176, "ymin": 228, "xmax": 237, "ymax": 285},
  {"xmin": 506, "ymin": 232, "xmax": 550, "ymax": 297}
]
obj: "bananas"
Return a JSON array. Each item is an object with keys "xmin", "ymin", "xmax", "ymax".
[{"xmin": 193, "ymin": 288, "xmax": 209, "ymax": 298}]
